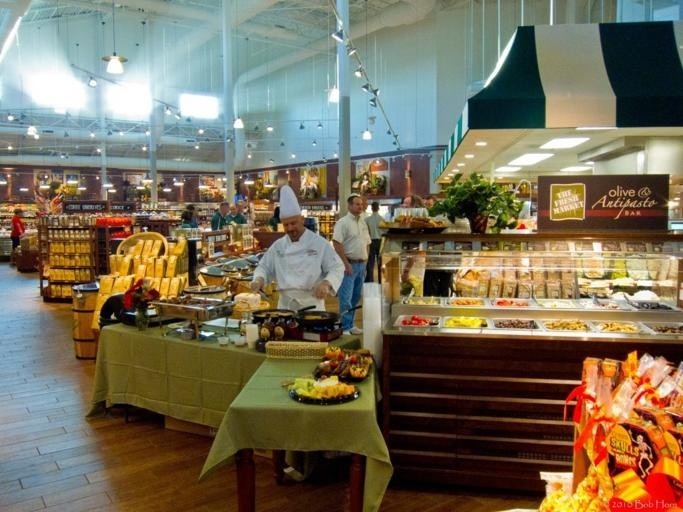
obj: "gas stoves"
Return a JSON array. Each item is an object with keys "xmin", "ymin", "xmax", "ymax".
[{"xmin": 257, "ymin": 323, "xmax": 344, "ymax": 342}]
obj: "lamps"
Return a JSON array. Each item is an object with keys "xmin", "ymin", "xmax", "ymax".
[
  {"xmin": 362, "ymin": 0, "xmax": 370, "ymax": 141},
  {"xmin": 232, "ymin": 3, "xmax": 244, "ymax": 129},
  {"xmin": 334, "ymin": 29, "xmax": 402, "ymax": 155},
  {"xmin": 102, "ymin": 1, "xmax": 128, "ymax": 74},
  {"xmin": 330, "ymin": 0, "xmax": 339, "ymax": 103}
]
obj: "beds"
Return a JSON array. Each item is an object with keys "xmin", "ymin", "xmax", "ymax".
[{"xmin": 199, "ymin": 357, "xmax": 394, "ymax": 512}]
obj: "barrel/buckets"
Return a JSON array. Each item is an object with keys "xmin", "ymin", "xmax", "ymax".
[{"xmin": 72, "ymin": 280, "xmax": 100, "ymax": 360}]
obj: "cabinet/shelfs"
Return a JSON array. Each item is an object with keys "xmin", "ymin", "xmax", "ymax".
[{"xmin": 383, "ymin": 229, "xmax": 683, "ymax": 493}]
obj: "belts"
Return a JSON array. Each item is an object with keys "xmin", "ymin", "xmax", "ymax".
[{"xmin": 346, "ymin": 260, "xmax": 367, "ymax": 263}]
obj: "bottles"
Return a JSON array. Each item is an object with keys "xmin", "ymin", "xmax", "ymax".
[
  {"xmin": 50, "ymin": 242, "xmax": 90, "ymax": 254},
  {"xmin": 49, "ymin": 253, "xmax": 91, "ymax": 267},
  {"xmin": 49, "ymin": 269, "xmax": 90, "ymax": 282},
  {"xmin": 51, "ymin": 285, "xmax": 72, "ymax": 298},
  {"xmin": 47, "ymin": 230, "xmax": 90, "ymax": 242},
  {"xmin": 47, "ymin": 215, "xmax": 96, "ymax": 226}
]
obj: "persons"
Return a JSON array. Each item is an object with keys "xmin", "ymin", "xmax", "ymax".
[
  {"xmin": 249, "ymin": 186, "xmax": 344, "ymax": 321},
  {"xmin": 384, "ymin": 194, "xmax": 438, "ymax": 225},
  {"xmin": 365, "ymin": 200, "xmax": 388, "ymax": 283},
  {"xmin": 332, "ymin": 192, "xmax": 371, "ymax": 336},
  {"xmin": 10, "ymin": 207, "xmax": 27, "ymax": 249},
  {"xmin": 270, "ymin": 207, "xmax": 281, "ymax": 230},
  {"xmin": 179, "ymin": 196, "xmax": 251, "ymax": 236}
]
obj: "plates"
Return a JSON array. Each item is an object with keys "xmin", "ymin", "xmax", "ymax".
[{"xmin": 176, "ymin": 328, "xmax": 194, "ymax": 335}]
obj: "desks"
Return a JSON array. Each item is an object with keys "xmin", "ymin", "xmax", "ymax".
[{"xmin": 85, "ymin": 317, "xmax": 360, "ymax": 464}]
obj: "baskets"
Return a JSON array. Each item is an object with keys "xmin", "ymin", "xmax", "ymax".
[
  {"xmin": 265, "ymin": 342, "xmax": 329, "ymax": 360},
  {"xmin": 232, "ymin": 300, "xmax": 271, "ymax": 320}
]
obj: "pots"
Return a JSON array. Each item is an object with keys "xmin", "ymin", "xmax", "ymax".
[
  {"xmin": 253, "ymin": 306, "xmax": 316, "ymax": 326},
  {"xmin": 295, "ymin": 305, "xmax": 361, "ymax": 326}
]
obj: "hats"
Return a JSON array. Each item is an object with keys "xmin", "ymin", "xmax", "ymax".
[{"xmin": 279, "ymin": 185, "xmax": 301, "ymax": 219}]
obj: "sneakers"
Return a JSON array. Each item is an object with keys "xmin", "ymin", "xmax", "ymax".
[{"xmin": 349, "ymin": 327, "xmax": 363, "ymax": 335}]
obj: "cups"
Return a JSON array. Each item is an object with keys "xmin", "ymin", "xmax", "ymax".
[{"xmin": 217, "ymin": 334, "xmax": 245, "ymax": 347}]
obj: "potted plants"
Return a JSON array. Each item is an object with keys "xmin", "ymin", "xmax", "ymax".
[{"xmin": 425, "ymin": 174, "xmax": 524, "ymax": 234}]
